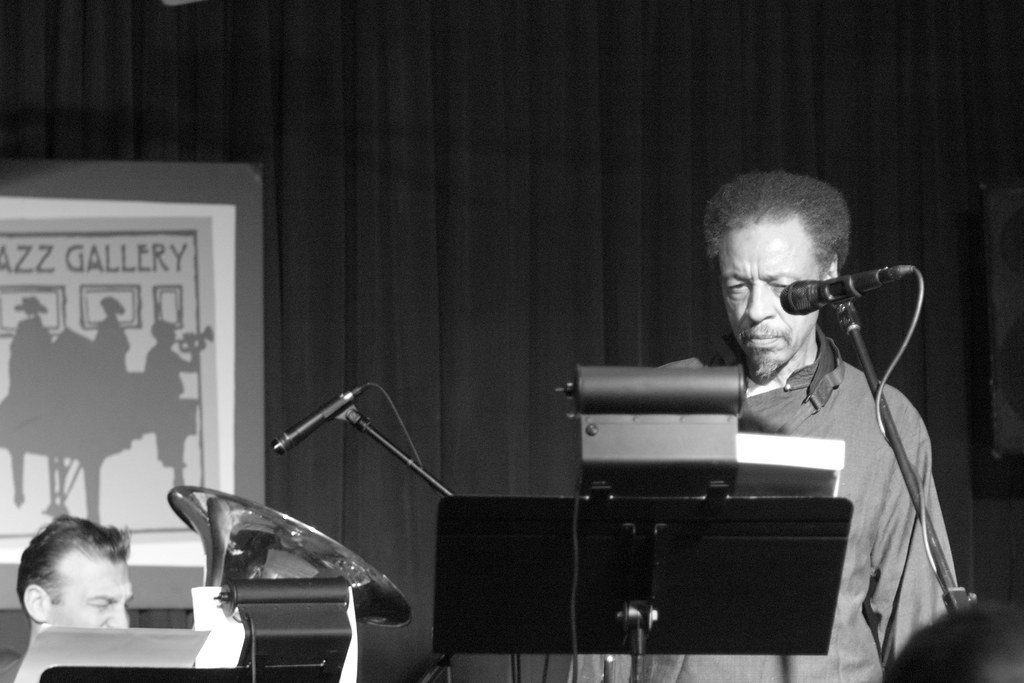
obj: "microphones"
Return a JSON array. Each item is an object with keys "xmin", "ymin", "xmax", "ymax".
[
  {"xmin": 781, "ymin": 264, "xmax": 914, "ymax": 316},
  {"xmin": 271, "ymin": 383, "xmax": 370, "ymax": 457}
]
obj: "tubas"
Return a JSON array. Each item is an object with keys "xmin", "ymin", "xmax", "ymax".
[{"xmin": 167, "ymin": 483, "xmax": 414, "ymax": 663}]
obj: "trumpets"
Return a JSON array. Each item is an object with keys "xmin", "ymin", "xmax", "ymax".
[{"xmin": 176, "ymin": 326, "xmax": 214, "ymax": 353}]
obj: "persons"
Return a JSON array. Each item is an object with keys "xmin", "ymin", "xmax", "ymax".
[
  {"xmin": 568, "ymin": 170, "xmax": 958, "ymax": 683},
  {"xmin": 16, "ymin": 514, "xmax": 134, "ymax": 645}
]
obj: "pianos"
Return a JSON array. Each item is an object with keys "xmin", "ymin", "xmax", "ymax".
[{"xmin": 0, "ymin": 330, "xmax": 181, "ymax": 528}]
